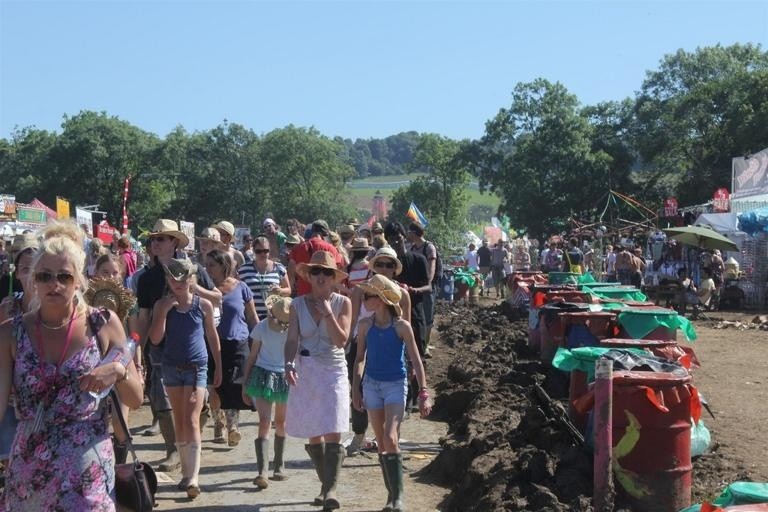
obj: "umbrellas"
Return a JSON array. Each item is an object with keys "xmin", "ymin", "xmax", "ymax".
[{"xmin": 663, "ymin": 224, "xmax": 738, "ymax": 279}]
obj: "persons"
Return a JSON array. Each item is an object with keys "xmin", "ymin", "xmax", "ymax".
[
  {"xmin": 676, "ymin": 268, "xmax": 716, "ymax": 316},
  {"xmin": 704, "ymin": 248, "xmax": 725, "ymax": 310},
  {"xmin": 0, "ymin": 217, "xmax": 438, "ymax": 509},
  {"xmin": 465, "ymin": 235, "xmax": 648, "ymax": 301}
]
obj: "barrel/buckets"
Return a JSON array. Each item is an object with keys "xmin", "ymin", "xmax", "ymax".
[
  {"xmin": 548, "ymin": 272, "xmax": 581, "ymax": 286},
  {"xmin": 546, "ymin": 290, "xmax": 592, "ymax": 304},
  {"xmin": 599, "ymin": 299, "xmax": 656, "ymax": 309},
  {"xmin": 506, "ymin": 273, "xmax": 514, "ymax": 305},
  {"xmin": 454, "ymin": 271, "xmax": 471, "ymax": 305},
  {"xmin": 619, "ymin": 309, "xmax": 676, "ymax": 338},
  {"xmin": 569, "ymin": 347, "xmax": 647, "ymax": 437},
  {"xmin": 447, "ymin": 254, "xmax": 467, "ymax": 269},
  {"xmin": 512, "ymin": 270, "xmax": 543, "ymax": 309},
  {"xmin": 729, "ymin": 481, "xmax": 768, "ymax": 505},
  {"xmin": 528, "ymin": 285, "xmax": 573, "ymax": 352},
  {"xmin": 722, "ymin": 502, "xmax": 768, "ymax": 512},
  {"xmin": 600, "ymin": 337, "xmax": 677, "ymax": 358},
  {"xmin": 469, "ymin": 272, "xmax": 479, "ymax": 303},
  {"xmin": 591, "ymin": 288, "xmax": 640, "ymax": 300},
  {"xmin": 439, "ymin": 268, "xmax": 454, "ymax": 301},
  {"xmin": 609, "ymin": 370, "xmax": 692, "ymax": 512},
  {"xmin": 538, "ymin": 303, "xmax": 589, "ymax": 379},
  {"xmin": 558, "ymin": 312, "xmax": 617, "ymax": 351},
  {"xmin": 579, "ymin": 282, "xmax": 622, "ymax": 290}
]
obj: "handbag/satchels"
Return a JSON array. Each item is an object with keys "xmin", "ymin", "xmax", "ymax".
[
  {"xmin": 570, "ymin": 265, "xmax": 581, "ymax": 273},
  {"xmin": 115, "ymin": 463, "xmax": 157, "ymax": 511}
]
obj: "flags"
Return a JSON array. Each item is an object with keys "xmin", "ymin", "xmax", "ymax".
[{"xmin": 406, "ymin": 200, "xmax": 428, "ymax": 230}]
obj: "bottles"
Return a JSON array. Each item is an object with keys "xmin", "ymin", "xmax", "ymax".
[{"xmin": 89, "ymin": 331, "xmax": 140, "ymax": 398}]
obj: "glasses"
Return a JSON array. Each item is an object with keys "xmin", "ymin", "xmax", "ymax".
[
  {"xmin": 364, "ymin": 292, "xmax": 378, "ymax": 300},
  {"xmin": 254, "ymin": 249, "xmax": 270, "ymax": 254},
  {"xmin": 151, "ymin": 235, "xmax": 169, "ymax": 242},
  {"xmin": 265, "ymin": 224, "xmax": 274, "ymax": 229},
  {"xmin": 311, "ymin": 266, "xmax": 334, "ymax": 276},
  {"xmin": 375, "ymin": 261, "xmax": 395, "ymax": 268},
  {"xmin": 34, "ymin": 271, "xmax": 73, "ymax": 285}
]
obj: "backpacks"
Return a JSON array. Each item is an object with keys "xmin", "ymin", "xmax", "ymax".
[{"xmin": 412, "ymin": 241, "xmax": 443, "ymax": 279}]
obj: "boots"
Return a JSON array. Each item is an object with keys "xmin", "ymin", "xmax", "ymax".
[
  {"xmin": 212, "ymin": 407, "xmax": 241, "ymax": 446},
  {"xmin": 378, "ymin": 453, "xmax": 406, "ymax": 511},
  {"xmin": 273, "ymin": 434, "xmax": 289, "ymax": 480},
  {"xmin": 158, "ymin": 407, "xmax": 182, "ymax": 472},
  {"xmin": 306, "ymin": 441, "xmax": 345, "ymax": 511},
  {"xmin": 253, "ymin": 439, "xmax": 269, "ymax": 487},
  {"xmin": 143, "ymin": 389, "xmax": 162, "ymax": 436},
  {"xmin": 112, "ymin": 434, "xmax": 131, "ymax": 461}
]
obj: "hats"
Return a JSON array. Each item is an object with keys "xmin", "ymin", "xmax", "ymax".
[
  {"xmin": 265, "ymin": 295, "xmax": 292, "ymax": 332},
  {"xmin": 196, "ymin": 220, "xmax": 238, "ymax": 247},
  {"xmin": 12, "ymin": 235, "xmax": 42, "ymax": 261},
  {"xmin": 483, "ymin": 239, "xmax": 488, "ymax": 243},
  {"xmin": 355, "ymin": 273, "xmax": 403, "ymax": 317},
  {"xmin": 349, "ymin": 237, "xmax": 374, "ymax": 251},
  {"xmin": 163, "ymin": 258, "xmax": 198, "ymax": 282},
  {"xmin": 284, "ymin": 233, "xmax": 301, "ymax": 243},
  {"xmin": 368, "ymin": 248, "xmax": 402, "ymax": 277},
  {"xmin": 264, "ymin": 218, "xmax": 275, "ymax": 226},
  {"xmin": 372, "ymin": 222, "xmax": 384, "ymax": 231},
  {"xmin": 296, "ymin": 250, "xmax": 349, "ymax": 285},
  {"xmin": 150, "ymin": 219, "xmax": 189, "ymax": 250},
  {"xmin": 340, "ymin": 216, "xmax": 360, "ymax": 234},
  {"xmin": 86, "ymin": 276, "xmax": 134, "ymax": 323}
]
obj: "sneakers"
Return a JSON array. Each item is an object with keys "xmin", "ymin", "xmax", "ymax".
[
  {"xmin": 479, "ymin": 289, "xmax": 502, "ymax": 300},
  {"xmin": 423, "ymin": 345, "xmax": 435, "ymax": 358},
  {"xmin": 347, "ymin": 435, "xmax": 365, "ymax": 455},
  {"xmin": 177, "ymin": 477, "xmax": 201, "ymax": 499}
]
